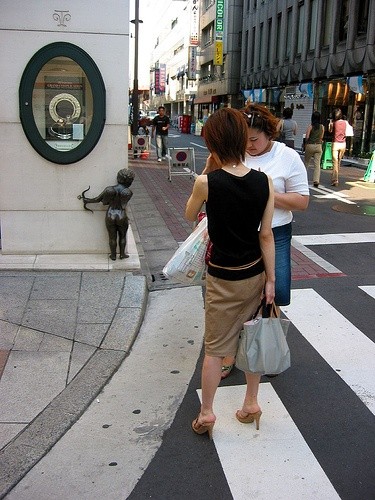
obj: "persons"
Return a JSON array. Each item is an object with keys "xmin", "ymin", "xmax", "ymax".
[
  {"xmin": 152, "ymin": 106, "xmax": 170, "ymax": 162},
  {"xmin": 220, "ymin": 104, "xmax": 309, "ymax": 377},
  {"xmin": 184, "ymin": 107, "xmax": 276, "ymax": 430},
  {"xmin": 275, "ymin": 107, "xmax": 297, "ymax": 149},
  {"xmin": 304, "ymin": 111, "xmax": 325, "ymax": 188},
  {"xmin": 328, "ymin": 109, "xmax": 351, "ymax": 186},
  {"xmin": 85, "ymin": 167, "xmax": 135, "ymax": 261}
]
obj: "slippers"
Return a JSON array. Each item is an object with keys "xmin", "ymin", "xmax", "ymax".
[{"xmin": 221, "ymin": 356, "xmax": 236, "ymax": 377}]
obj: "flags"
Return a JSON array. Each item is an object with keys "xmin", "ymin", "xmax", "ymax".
[
  {"xmin": 347, "ymin": 76, "xmax": 365, "ymax": 94},
  {"xmin": 251, "ymin": 88, "xmax": 267, "ymax": 103},
  {"xmin": 299, "ymin": 82, "xmax": 313, "ymax": 99},
  {"xmin": 241, "ymin": 89, "xmax": 251, "ymax": 99}
]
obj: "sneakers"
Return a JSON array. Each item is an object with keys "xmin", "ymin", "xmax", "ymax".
[
  {"xmin": 314, "ymin": 182, "xmax": 319, "ymax": 188},
  {"xmin": 158, "ymin": 158, "xmax": 162, "ymax": 162},
  {"xmin": 162, "ymin": 156, "xmax": 166, "ymax": 160}
]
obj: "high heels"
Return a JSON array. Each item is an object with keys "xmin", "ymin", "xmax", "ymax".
[
  {"xmin": 236, "ymin": 409, "xmax": 262, "ymax": 430},
  {"xmin": 191, "ymin": 417, "xmax": 215, "ymax": 441},
  {"xmin": 330, "ymin": 181, "xmax": 338, "ymax": 187}
]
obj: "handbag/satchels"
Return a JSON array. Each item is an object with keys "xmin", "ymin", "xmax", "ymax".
[
  {"xmin": 344, "ymin": 121, "xmax": 354, "ymax": 136},
  {"xmin": 236, "ymin": 297, "xmax": 292, "ymax": 375},
  {"xmin": 278, "ymin": 120, "xmax": 286, "ymax": 143}
]
